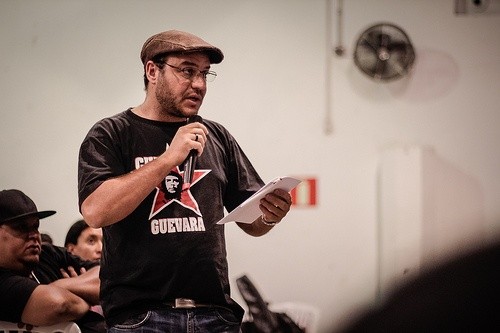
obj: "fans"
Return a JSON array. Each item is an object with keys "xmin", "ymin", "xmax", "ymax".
[{"xmin": 353, "ymin": 23, "xmax": 416, "ymax": 82}]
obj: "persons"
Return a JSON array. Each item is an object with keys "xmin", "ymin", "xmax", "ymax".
[
  {"xmin": 77, "ymin": 28, "xmax": 293, "ymax": 333},
  {"xmin": 0, "ymin": 189, "xmax": 105, "ymax": 333}
]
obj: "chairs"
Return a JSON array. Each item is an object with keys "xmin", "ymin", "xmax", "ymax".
[{"xmin": 236, "ymin": 275, "xmax": 306, "ymax": 333}]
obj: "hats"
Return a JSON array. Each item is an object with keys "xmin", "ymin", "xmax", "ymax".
[
  {"xmin": 141, "ymin": 31, "xmax": 224, "ymax": 62},
  {"xmin": 0, "ymin": 189, "xmax": 56, "ymax": 223}
]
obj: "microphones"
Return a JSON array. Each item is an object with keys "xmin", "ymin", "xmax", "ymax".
[{"xmin": 182, "ymin": 115, "xmax": 204, "ymax": 190}]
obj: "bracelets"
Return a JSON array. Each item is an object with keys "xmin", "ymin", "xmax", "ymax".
[{"xmin": 260, "ymin": 214, "xmax": 282, "ymax": 226}]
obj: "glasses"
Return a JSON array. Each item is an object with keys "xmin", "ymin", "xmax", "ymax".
[{"xmin": 162, "ymin": 63, "xmax": 216, "ymax": 81}]
